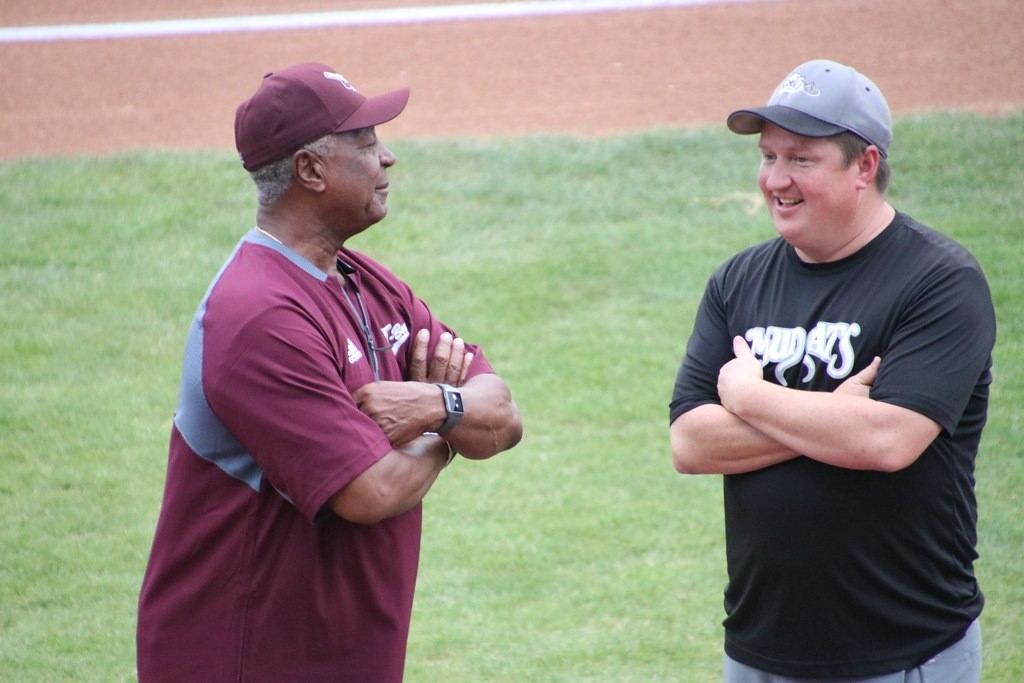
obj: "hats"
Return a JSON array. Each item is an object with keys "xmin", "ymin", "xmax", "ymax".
[
  {"xmin": 234, "ymin": 62, "xmax": 411, "ymax": 170},
  {"xmin": 726, "ymin": 59, "xmax": 893, "ymax": 161}
]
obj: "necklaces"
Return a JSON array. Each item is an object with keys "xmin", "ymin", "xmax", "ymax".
[{"xmin": 256, "ymin": 226, "xmax": 283, "ymax": 244}]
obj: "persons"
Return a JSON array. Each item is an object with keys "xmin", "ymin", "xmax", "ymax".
[
  {"xmin": 136, "ymin": 62, "xmax": 523, "ymax": 683},
  {"xmin": 668, "ymin": 59, "xmax": 997, "ymax": 683}
]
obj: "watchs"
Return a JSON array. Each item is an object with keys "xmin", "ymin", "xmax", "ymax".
[{"xmin": 436, "ymin": 383, "xmax": 464, "ymax": 434}]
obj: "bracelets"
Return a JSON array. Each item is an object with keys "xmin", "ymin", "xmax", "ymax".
[{"xmin": 423, "ymin": 432, "xmax": 458, "ymax": 465}]
